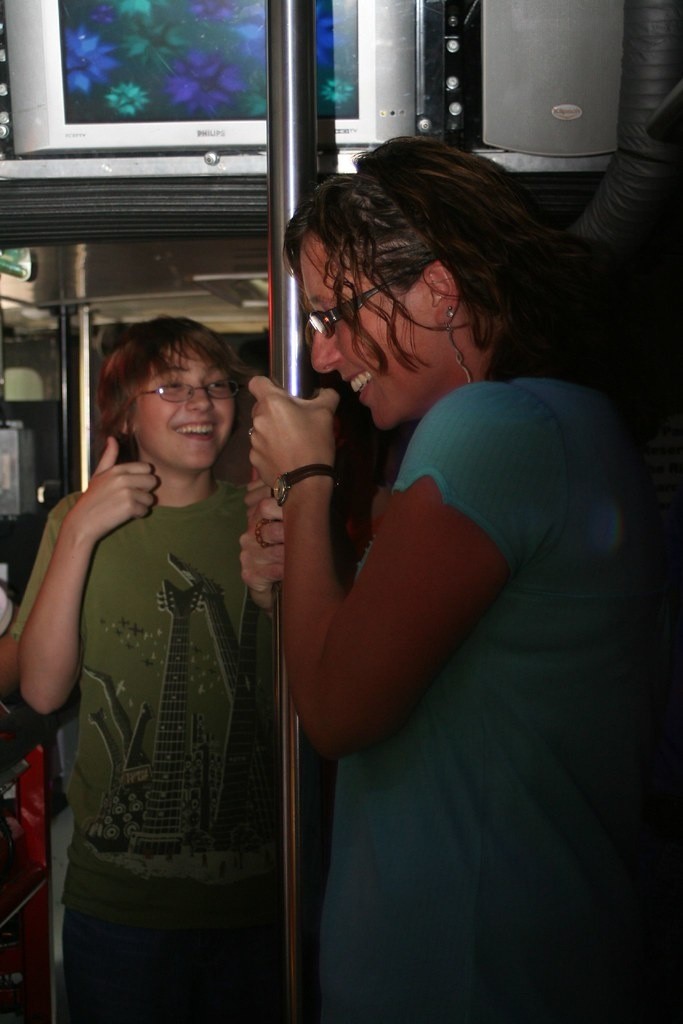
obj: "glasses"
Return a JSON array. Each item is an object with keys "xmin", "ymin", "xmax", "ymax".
[
  {"xmin": 310, "ymin": 280, "xmax": 399, "ymax": 338},
  {"xmin": 140, "ymin": 379, "xmax": 239, "ymax": 403}
]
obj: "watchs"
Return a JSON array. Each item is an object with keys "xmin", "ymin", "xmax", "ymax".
[{"xmin": 273, "ymin": 465, "xmax": 341, "ymax": 506}]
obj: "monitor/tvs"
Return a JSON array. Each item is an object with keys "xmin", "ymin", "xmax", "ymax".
[{"xmin": 0, "ymin": 0, "xmax": 419, "ymax": 181}]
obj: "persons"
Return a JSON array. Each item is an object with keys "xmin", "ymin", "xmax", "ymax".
[
  {"xmin": 238, "ymin": 137, "xmax": 683, "ymax": 1024},
  {"xmin": 0, "ymin": 564, "xmax": 26, "ymax": 694},
  {"xmin": 11, "ymin": 317, "xmax": 332, "ymax": 1023}
]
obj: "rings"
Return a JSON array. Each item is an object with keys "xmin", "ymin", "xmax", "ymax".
[{"xmin": 255, "ymin": 519, "xmax": 270, "ymax": 547}]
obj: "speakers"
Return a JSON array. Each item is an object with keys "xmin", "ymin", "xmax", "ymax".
[{"xmin": 477, "ymin": 0, "xmax": 626, "ymax": 157}]
